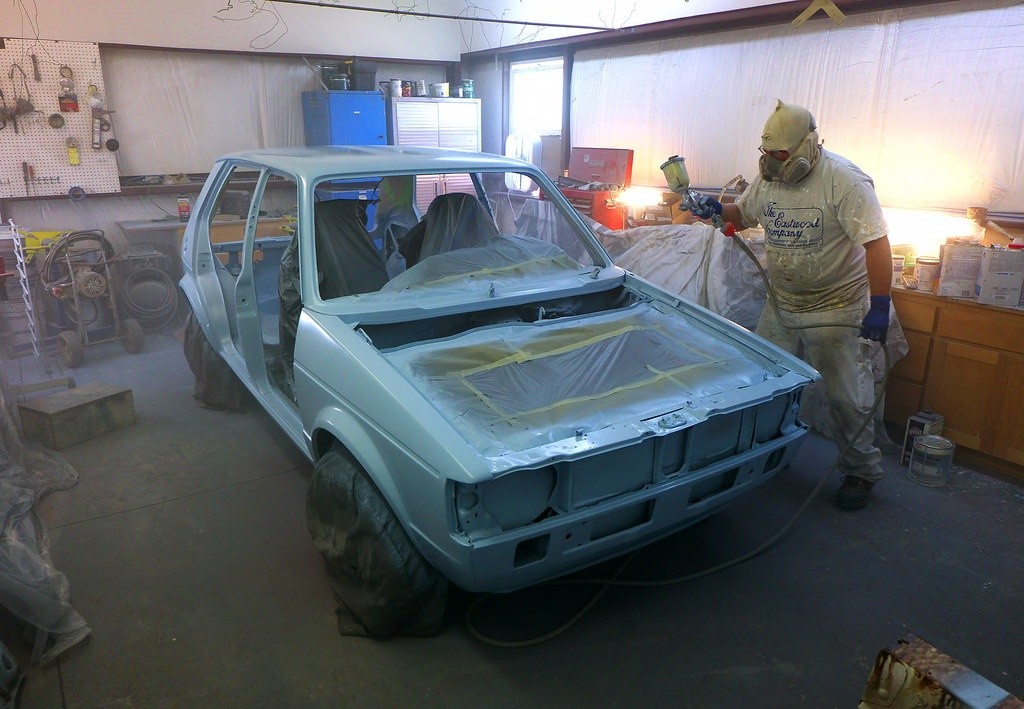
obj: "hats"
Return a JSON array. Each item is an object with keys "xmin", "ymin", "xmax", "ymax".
[{"xmin": 760, "ymin": 102, "xmax": 816, "ymax": 155}]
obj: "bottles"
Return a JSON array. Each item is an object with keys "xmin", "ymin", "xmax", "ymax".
[
  {"xmin": 177, "ymin": 192, "xmax": 191, "ymax": 222},
  {"xmin": 379, "ymin": 76, "xmax": 474, "ymax": 99}
]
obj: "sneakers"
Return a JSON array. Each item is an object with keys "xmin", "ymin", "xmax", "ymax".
[{"xmin": 836, "ymin": 476, "xmax": 874, "ymax": 509}]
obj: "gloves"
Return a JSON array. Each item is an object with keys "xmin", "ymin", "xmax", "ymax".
[
  {"xmin": 689, "ymin": 195, "xmax": 723, "ymax": 220},
  {"xmin": 856, "ymin": 295, "xmax": 890, "ymax": 344}
]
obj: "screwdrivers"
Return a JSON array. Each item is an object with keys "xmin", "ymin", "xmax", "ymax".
[
  {"xmin": 21, "ymin": 162, "xmax": 29, "ymax": 197},
  {"xmin": 29, "ymin": 165, "xmax": 38, "ymax": 197}
]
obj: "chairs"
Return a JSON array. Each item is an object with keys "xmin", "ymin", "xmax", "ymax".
[{"xmin": 421, "ymin": 193, "xmax": 500, "ymax": 265}]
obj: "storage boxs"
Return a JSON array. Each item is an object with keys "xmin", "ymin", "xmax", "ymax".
[
  {"xmin": 17, "ymin": 383, "xmax": 137, "ymax": 452},
  {"xmin": 645, "ymin": 191, "xmax": 691, "ymax": 228},
  {"xmin": 320, "ymin": 59, "xmax": 377, "ymax": 91}
]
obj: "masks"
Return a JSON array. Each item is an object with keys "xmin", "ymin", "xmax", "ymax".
[{"xmin": 758, "ymin": 127, "xmax": 819, "ymax": 184}]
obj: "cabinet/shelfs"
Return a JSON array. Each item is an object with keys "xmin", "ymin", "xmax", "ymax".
[
  {"xmin": 920, "ymin": 305, "xmax": 1024, "ymax": 483},
  {"xmin": 871, "ymin": 289, "xmax": 940, "ymax": 442},
  {"xmin": 387, "ymin": 96, "xmax": 482, "ymax": 217},
  {"xmin": 315, "ymin": 187, "xmax": 379, "ymax": 232},
  {"xmin": 175, "ymin": 220, "xmax": 292, "ymax": 267},
  {"xmin": 301, "ymin": 89, "xmax": 388, "ymax": 184}
]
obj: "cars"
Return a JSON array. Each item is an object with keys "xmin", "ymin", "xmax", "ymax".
[{"xmin": 178, "ymin": 144, "xmax": 822, "ymax": 637}]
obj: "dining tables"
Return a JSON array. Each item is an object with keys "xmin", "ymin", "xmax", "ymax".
[{"xmin": 280, "ymin": 196, "xmax": 390, "ymax": 392}]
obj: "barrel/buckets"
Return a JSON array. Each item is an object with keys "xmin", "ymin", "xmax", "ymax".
[
  {"xmin": 890, "ymin": 253, "xmax": 939, "ymax": 292},
  {"xmin": 908, "ymin": 434, "xmax": 955, "ymax": 488},
  {"xmin": 901, "ymin": 409, "xmax": 944, "ymax": 465}
]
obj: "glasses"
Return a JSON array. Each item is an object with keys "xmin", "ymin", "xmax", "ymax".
[{"xmin": 758, "ymin": 145, "xmax": 789, "ymax": 160}]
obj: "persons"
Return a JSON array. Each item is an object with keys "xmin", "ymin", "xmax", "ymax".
[{"xmin": 691, "ymin": 98, "xmax": 895, "ymax": 512}]
